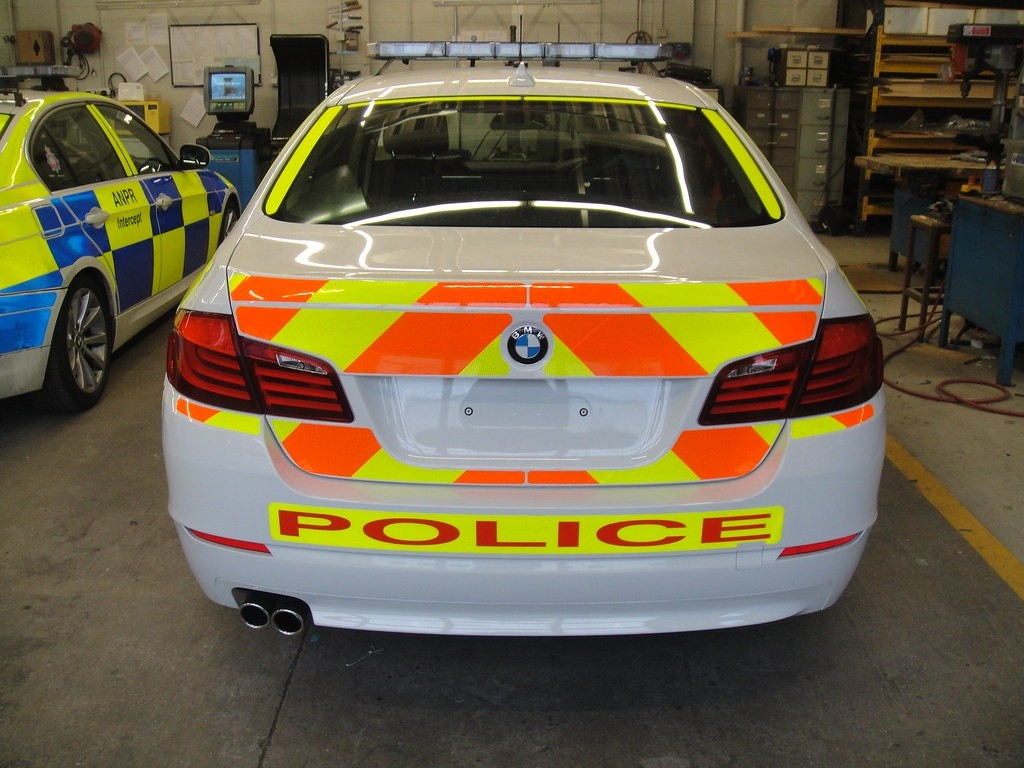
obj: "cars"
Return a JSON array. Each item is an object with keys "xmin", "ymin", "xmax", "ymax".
[
  {"xmin": 0, "ymin": 82, "xmax": 243, "ymax": 414},
  {"xmin": 161, "ymin": 68, "xmax": 884, "ymax": 636}
]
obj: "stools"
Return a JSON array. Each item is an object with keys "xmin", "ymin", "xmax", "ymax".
[{"xmin": 897, "ymin": 214, "xmax": 953, "ymax": 342}]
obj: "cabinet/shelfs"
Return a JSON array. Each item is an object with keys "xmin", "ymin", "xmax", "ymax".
[
  {"xmin": 732, "ymin": 85, "xmax": 801, "ymax": 207},
  {"xmin": 796, "ymin": 85, "xmax": 852, "ymax": 228},
  {"xmin": 852, "ymin": 25, "xmax": 1024, "ymax": 240}
]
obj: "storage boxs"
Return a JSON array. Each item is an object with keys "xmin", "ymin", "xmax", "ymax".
[
  {"xmin": 926, "ymin": 8, "xmax": 975, "ymax": 36},
  {"xmin": 774, "ymin": 48, "xmax": 830, "ymax": 88},
  {"xmin": 866, "ymin": 7, "xmax": 928, "ymax": 35},
  {"xmin": 974, "ymin": 9, "xmax": 1024, "ymax": 26}
]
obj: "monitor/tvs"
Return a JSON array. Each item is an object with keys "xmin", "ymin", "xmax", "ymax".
[{"xmin": 203, "ymin": 66, "xmax": 256, "ymax": 113}]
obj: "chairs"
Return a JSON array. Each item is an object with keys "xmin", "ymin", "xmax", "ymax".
[{"xmin": 365, "ymin": 110, "xmax": 484, "ymax": 209}]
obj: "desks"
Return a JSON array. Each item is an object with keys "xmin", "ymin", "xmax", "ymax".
[
  {"xmin": 853, "ymin": 154, "xmax": 1007, "ymax": 272},
  {"xmin": 937, "ymin": 194, "xmax": 1024, "ymax": 389}
]
